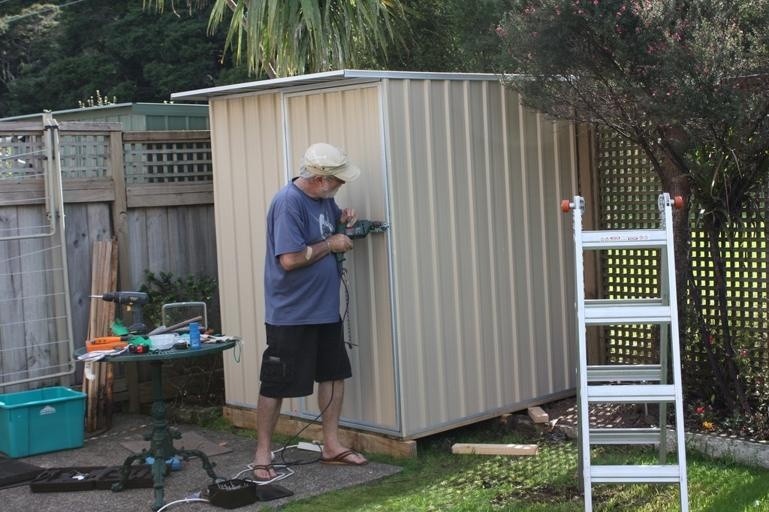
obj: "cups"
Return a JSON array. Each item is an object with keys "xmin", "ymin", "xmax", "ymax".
[{"xmin": 174, "ymin": 340, "xmax": 187, "ymax": 350}]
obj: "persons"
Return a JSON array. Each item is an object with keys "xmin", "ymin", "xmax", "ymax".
[{"xmin": 248, "ymin": 140, "xmax": 367, "ymax": 481}]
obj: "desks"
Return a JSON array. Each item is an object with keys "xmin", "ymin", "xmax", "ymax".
[{"xmin": 73, "ymin": 334, "xmax": 237, "ymax": 510}]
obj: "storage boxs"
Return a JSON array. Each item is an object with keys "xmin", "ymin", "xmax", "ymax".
[{"xmin": 0, "ymin": 386, "xmax": 88, "ymax": 458}]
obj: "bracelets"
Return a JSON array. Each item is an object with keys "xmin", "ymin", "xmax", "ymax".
[{"xmin": 323, "ymin": 240, "xmax": 332, "ymax": 256}]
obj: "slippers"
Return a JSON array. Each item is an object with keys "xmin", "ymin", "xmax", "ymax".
[
  {"xmin": 319, "ymin": 447, "xmax": 368, "ymax": 466},
  {"xmin": 253, "ymin": 462, "xmax": 278, "ymax": 481}
]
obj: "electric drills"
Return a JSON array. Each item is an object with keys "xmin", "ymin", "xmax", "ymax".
[
  {"xmin": 87, "ymin": 291, "xmax": 151, "ymax": 335},
  {"xmin": 335, "ymin": 219, "xmax": 390, "ymax": 260}
]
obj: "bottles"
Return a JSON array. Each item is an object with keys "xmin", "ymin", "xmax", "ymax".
[{"xmin": 190, "ymin": 322, "xmax": 202, "ymax": 350}]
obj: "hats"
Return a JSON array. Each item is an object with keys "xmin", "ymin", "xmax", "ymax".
[{"xmin": 304, "ymin": 142, "xmax": 361, "ymax": 183}]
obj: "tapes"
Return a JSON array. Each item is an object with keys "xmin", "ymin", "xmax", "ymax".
[{"xmin": 175, "ymin": 343, "xmax": 187, "ymax": 349}]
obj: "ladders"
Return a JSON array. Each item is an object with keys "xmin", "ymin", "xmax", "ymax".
[{"xmin": 559, "ymin": 193, "xmax": 689, "ymax": 511}]
